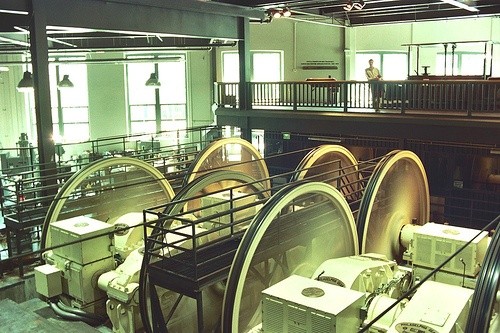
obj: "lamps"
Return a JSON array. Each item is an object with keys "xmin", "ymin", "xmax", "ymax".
[
  {"xmin": 58, "ymin": 73, "xmax": 75, "ymax": 90},
  {"xmin": 15, "ymin": 50, "xmax": 34, "ymax": 93},
  {"xmin": 271, "ymin": 7, "xmax": 292, "ymax": 18},
  {"xmin": 343, "ymin": 0, "xmax": 367, "ymax": 11},
  {"xmin": 144, "ymin": 73, "xmax": 162, "ymax": 89}
]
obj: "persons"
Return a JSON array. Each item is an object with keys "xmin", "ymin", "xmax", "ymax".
[{"xmin": 365, "ymin": 58, "xmax": 385, "ymax": 108}]
